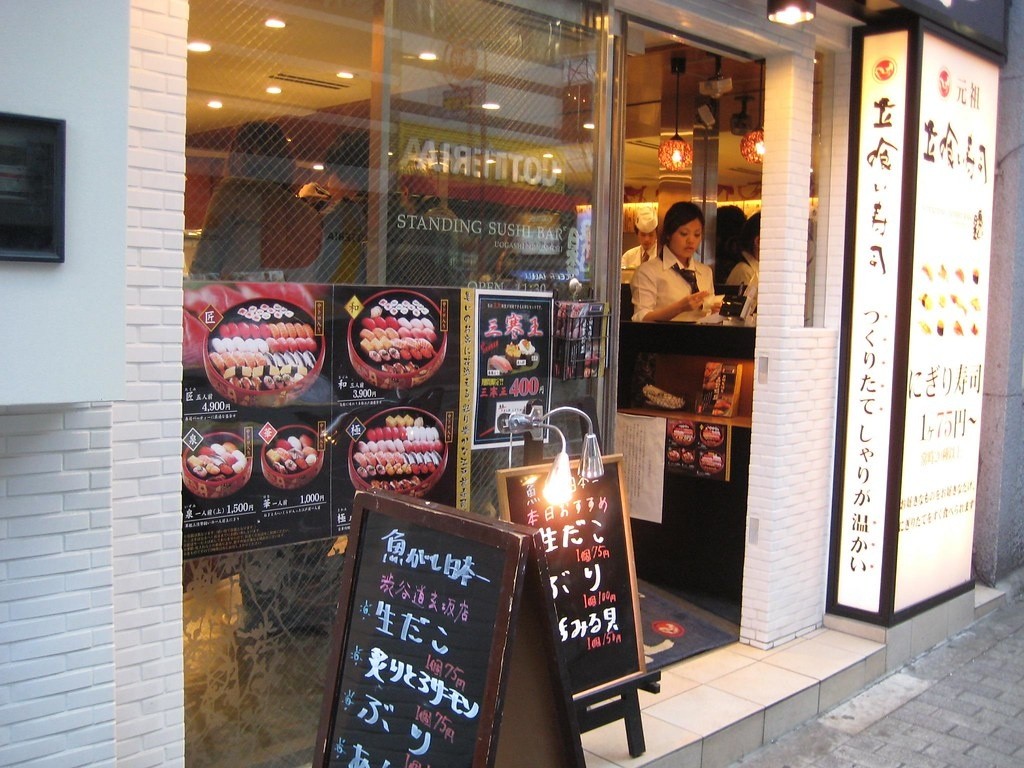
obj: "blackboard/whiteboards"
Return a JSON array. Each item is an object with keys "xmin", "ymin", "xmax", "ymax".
[
  {"xmin": 311, "ymin": 487, "xmax": 586, "ymax": 768},
  {"xmin": 495, "ymin": 453, "xmax": 648, "ymax": 700}
]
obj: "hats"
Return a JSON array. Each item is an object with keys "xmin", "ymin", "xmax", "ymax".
[{"xmin": 634, "ymin": 206, "xmax": 659, "ymax": 233}]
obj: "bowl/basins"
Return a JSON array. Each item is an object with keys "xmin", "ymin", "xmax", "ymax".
[
  {"xmin": 182, "ymin": 432, "xmax": 252, "ymax": 498},
  {"xmin": 260, "ymin": 425, "xmax": 323, "ymax": 489},
  {"xmin": 203, "ymin": 298, "xmax": 326, "ymax": 408},
  {"xmin": 699, "ymin": 450, "xmax": 724, "ymax": 474},
  {"xmin": 700, "ymin": 425, "xmax": 724, "ymax": 448},
  {"xmin": 672, "ymin": 423, "xmax": 695, "ymax": 447},
  {"xmin": 667, "ymin": 449, "xmax": 681, "ymax": 462},
  {"xmin": 348, "ymin": 407, "xmax": 448, "ymax": 497},
  {"xmin": 347, "ymin": 289, "xmax": 448, "ymax": 390},
  {"xmin": 682, "ymin": 451, "xmax": 694, "ymax": 463}
]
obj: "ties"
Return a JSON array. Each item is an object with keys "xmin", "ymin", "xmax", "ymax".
[
  {"xmin": 673, "ymin": 264, "xmax": 703, "ymax": 309},
  {"xmin": 644, "ymin": 250, "xmax": 649, "ymax": 263}
]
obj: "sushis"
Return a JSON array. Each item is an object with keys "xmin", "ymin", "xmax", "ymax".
[
  {"xmin": 265, "ymin": 434, "xmax": 318, "ymax": 473},
  {"xmin": 187, "ymin": 441, "xmax": 247, "ymax": 477},
  {"xmin": 353, "ymin": 414, "xmax": 443, "ymax": 490},
  {"xmin": 360, "ymin": 298, "xmax": 438, "ymax": 373},
  {"xmin": 208, "ymin": 303, "xmax": 317, "ymax": 391},
  {"xmin": 489, "ymin": 339, "xmax": 536, "ymax": 374}
]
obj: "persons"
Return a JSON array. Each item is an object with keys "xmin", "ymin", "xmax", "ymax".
[
  {"xmin": 621, "ymin": 204, "xmax": 660, "ymax": 269},
  {"xmin": 398, "ymin": 154, "xmax": 473, "ymax": 285},
  {"xmin": 721, "ymin": 211, "xmax": 760, "ymax": 316},
  {"xmin": 310, "ymin": 128, "xmax": 411, "ymax": 288},
  {"xmin": 193, "ymin": 118, "xmax": 320, "ymax": 285},
  {"xmin": 629, "ymin": 200, "xmax": 721, "ymax": 323}
]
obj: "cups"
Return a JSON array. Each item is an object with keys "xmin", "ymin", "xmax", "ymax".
[{"xmin": 309, "ymin": 187, "xmax": 331, "ymax": 212}]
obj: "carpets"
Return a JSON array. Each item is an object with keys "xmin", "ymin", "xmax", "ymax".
[{"xmin": 637, "ymin": 586, "xmax": 739, "ymax": 672}]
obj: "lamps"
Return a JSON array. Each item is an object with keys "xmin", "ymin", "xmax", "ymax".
[
  {"xmin": 740, "ymin": 59, "xmax": 763, "ymax": 163},
  {"xmin": 659, "ymin": 56, "xmax": 693, "ymax": 173},
  {"xmin": 729, "ymin": 96, "xmax": 755, "ymax": 135},
  {"xmin": 499, "ymin": 404, "xmax": 605, "ymax": 506},
  {"xmin": 766, "ymin": 0, "xmax": 816, "ymax": 24}
]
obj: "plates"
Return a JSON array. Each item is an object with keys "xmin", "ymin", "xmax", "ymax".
[{"xmin": 487, "ymin": 353, "xmax": 540, "ymax": 376}]
